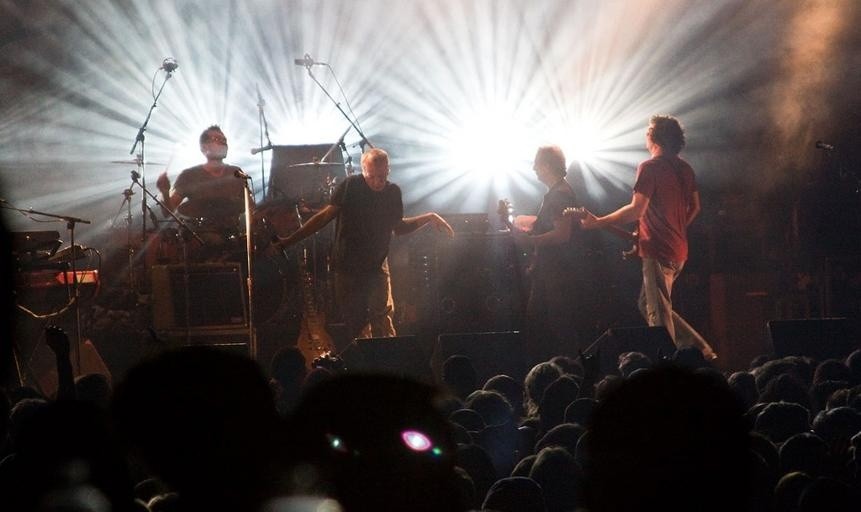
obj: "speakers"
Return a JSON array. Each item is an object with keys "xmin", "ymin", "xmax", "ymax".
[
  {"xmin": 0, "ymin": 328, "xmax": 112, "ymax": 404},
  {"xmin": 338, "ymin": 336, "xmax": 433, "ymax": 385},
  {"xmin": 433, "ymin": 331, "xmax": 526, "ymax": 395},
  {"xmin": 574, "ymin": 326, "xmax": 678, "ymax": 383},
  {"xmin": 762, "ymin": 317, "xmax": 861, "ymax": 370}
]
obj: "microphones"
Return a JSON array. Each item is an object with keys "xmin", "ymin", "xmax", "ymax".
[
  {"xmin": 164, "ymin": 63, "xmax": 177, "ymax": 70},
  {"xmin": 295, "ymin": 59, "xmax": 324, "ymax": 65},
  {"xmin": 251, "ymin": 145, "xmax": 275, "ymax": 154},
  {"xmin": 815, "ymin": 139, "xmax": 835, "ymax": 152},
  {"xmin": 233, "ymin": 168, "xmax": 249, "ymax": 180}
]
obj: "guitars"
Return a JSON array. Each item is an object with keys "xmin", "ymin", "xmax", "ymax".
[
  {"xmin": 294, "ymin": 272, "xmax": 336, "ymax": 370},
  {"xmin": 562, "ymin": 207, "xmax": 640, "ymax": 263}
]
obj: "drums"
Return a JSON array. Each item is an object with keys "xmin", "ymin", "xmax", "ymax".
[{"xmin": 146, "ymin": 227, "xmax": 180, "ymax": 265}]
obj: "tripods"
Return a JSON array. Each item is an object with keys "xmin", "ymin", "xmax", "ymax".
[
  {"xmin": 98, "ymin": 142, "xmax": 171, "ymax": 346},
  {"xmin": 106, "ymin": 199, "xmax": 152, "ymax": 312},
  {"xmin": 271, "ymin": 216, "xmax": 340, "ymax": 327}
]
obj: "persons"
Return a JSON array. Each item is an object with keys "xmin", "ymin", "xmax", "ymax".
[
  {"xmin": 272, "ymin": 149, "xmax": 453, "ymax": 340},
  {"xmin": 1, "ymin": 325, "xmax": 860, "ymax": 511},
  {"xmin": 581, "ymin": 116, "xmax": 700, "ymax": 344},
  {"xmin": 157, "ymin": 126, "xmax": 256, "ymax": 326},
  {"xmin": 511, "ymin": 148, "xmax": 578, "ymax": 367}
]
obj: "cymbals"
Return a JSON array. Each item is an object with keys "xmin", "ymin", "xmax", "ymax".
[
  {"xmin": 177, "ymin": 194, "xmax": 247, "ymax": 222},
  {"xmin": 290, "ymin": 157, "xmax": 344, "ymax": 168},
  {"xmin": 320, "ymin": 118, "xmax": 359, "ymax": 162}
]
